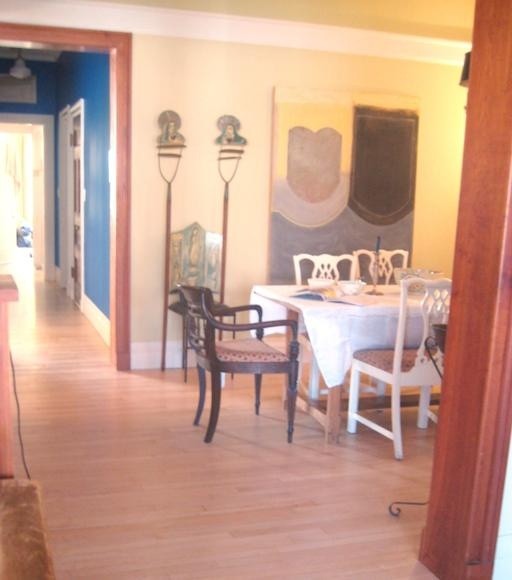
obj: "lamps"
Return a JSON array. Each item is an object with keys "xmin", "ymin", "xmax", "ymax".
[{"xmin": 8, "ymin": 49, "xmax": 31, "ymax": 80}]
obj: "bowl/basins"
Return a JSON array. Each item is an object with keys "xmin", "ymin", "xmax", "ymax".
[
  {"xmin": 307, "ymin": 278, "xmax": 366, "ymax": 295},
  {"xmin": 394, "ymin": 268, "xmax": 444, "ymax": 293}
]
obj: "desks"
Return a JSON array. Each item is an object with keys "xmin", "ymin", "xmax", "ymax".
[{"xmin": 251, "ymin": 284, "xmax": 452, "ymax": 446}]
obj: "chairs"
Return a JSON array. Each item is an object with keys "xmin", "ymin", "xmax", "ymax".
[
  {"xmin": 291, "ymin": 248, "xmax": 453, "ymax": 460},
  {"xmin": 168, "ymin": 283, "xmax": 299, "ymax": 444}
]
